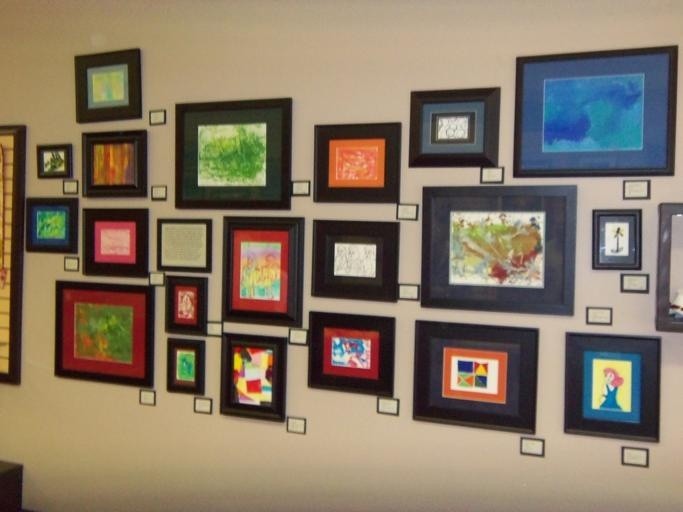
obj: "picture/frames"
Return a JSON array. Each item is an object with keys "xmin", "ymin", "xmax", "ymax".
[
  {"xmin": 165, "ymin": 276, "xmax": 208, "ymax": 336},
  {"xmin": 55, "ymin": 280, "xmax": 155, "ymax": 387},
  {"xmin": 27, "ymin": 197, "xmax": 79, "ymax": 254},
  {"xmin": 167, "ymin": 338, "xmax": 205, "ymax": 396},
  {"xmin": 157, "ymin": 219, "xmax": 212, "ymax": 273},
  {"xmin": 513, "ymin": 44, "xmax": 677, "ymax": 178},
  {"xmin": 174, "ymin": 97, "xmax": 293, "ymax": 211},
  {"xmin": 420, "ymin": 185, "xmax": 577, "ymax": 316},
  {"xmin": 591, "ymin": 209, "xmax": 642, "ymax": 270},
  {"xmin": 313, "ymin": 122, "xmax": 401, "ymax": 203},
  {"xmin": 75, "ymin": 48, "xmax": 143, "ymax": 124},
  {"xmin": 563, "ymin": 331, "xmax": 661, "ymax": 443},
  {"xmin": 311, "ymin": 220, "xmax": 400, "ymax": 303},
  {"xmin": 0, "ymin": 124, "xmax": 27, "ymax": 385},
  {"xmin": 221, "ymin": 216, "xmax": 306, "ymax": 328},
  {"xmin": 408, "ymin": 87, "xmax": 501, "ymax": 169},
  {"xmin": 412, "ymin": 319, "xmax": 539, "ymax": 435},
  {"xmin": 220, "ymin": 333, "xmax": 288, "ymax": 422},
  {"xmin": 307, "ymin": 310, "xmax": 396, "ymax": 398},
  {"xmin": 37, "ymin": 144, "xmax": 73, "ymax": 179},
  {"xmin": 82, "ymin": 129, "xmax": 149, "ymax": 198},
  {"xmin": 83, "ymin": 208, "xmax": 149, "ymax": 277},
  {"xmin": 654, "ymin": 202, "xmax": 682, "ymax": 333}
]
